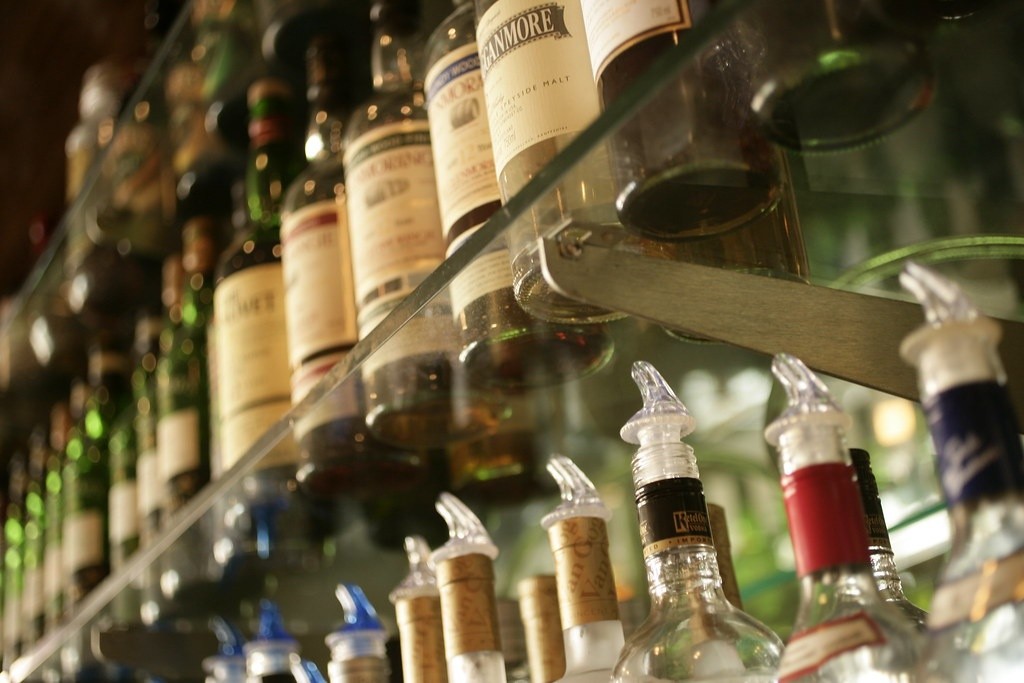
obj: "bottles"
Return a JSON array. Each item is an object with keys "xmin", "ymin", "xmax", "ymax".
[{"xmin": 1, "ymin": 0, "xmax": 1024, "ymax": 683}]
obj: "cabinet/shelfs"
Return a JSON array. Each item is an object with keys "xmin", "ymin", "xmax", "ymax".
[{"xmin": 0, "ymin": 0, "xmax": 1024, "ymax": 683}]
